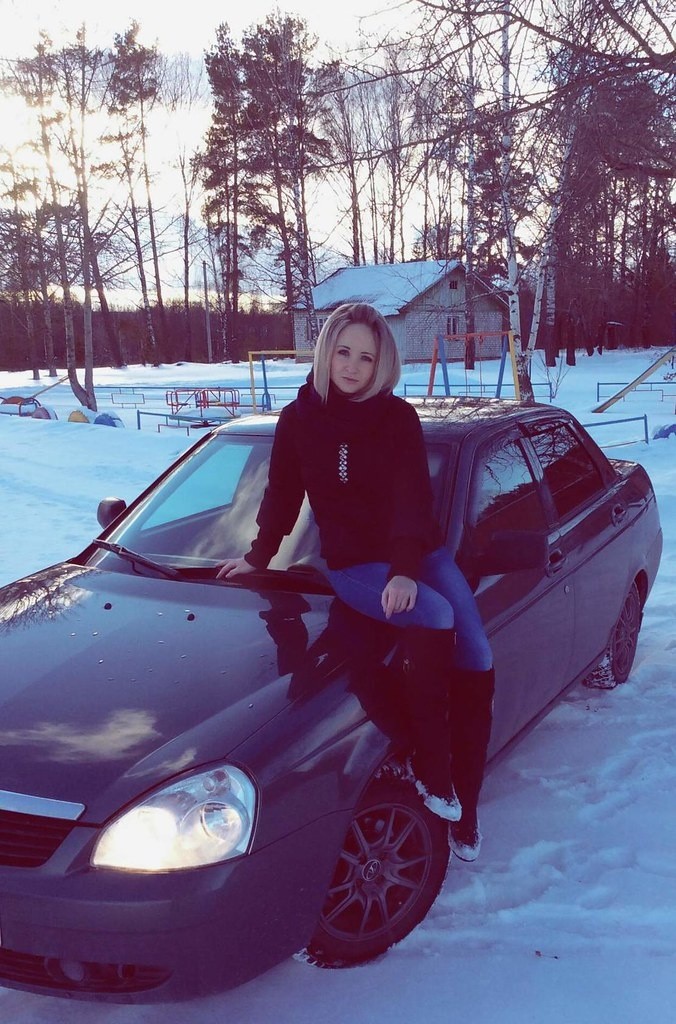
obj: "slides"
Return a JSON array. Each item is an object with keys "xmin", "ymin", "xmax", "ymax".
[{"xmin": 593, "ymin": 345, "xmax": 676, "ymax": 413}]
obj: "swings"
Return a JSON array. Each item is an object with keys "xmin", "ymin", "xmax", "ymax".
[{"xmin": 465, "ymin": 336, "xmax": 483, "ymax": 397}]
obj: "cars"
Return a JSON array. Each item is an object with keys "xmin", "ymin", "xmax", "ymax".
[{"xmin": 0, "ymin": 395, "xmax": 663, "ymax": 1004}]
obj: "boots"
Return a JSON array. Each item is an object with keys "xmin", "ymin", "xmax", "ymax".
[
  {"xmin": 446, "ymin": 665, "xmax": 496, "ymax": 862},
  {"xmin": 402, "ymin": 623, "xmax": 460, "ymax": 822}
]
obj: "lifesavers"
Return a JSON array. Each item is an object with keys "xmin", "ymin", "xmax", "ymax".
[
  {"xmin": 32, "ymin": 406, "xmax": 58, "ymax": 421},
  {"xmin": 92, "ymin": 411, "xmax": 125, "ymax": 428},
  {"xmin": 67, "ymin": 406, "xmax": 96, "ymax": 424}
]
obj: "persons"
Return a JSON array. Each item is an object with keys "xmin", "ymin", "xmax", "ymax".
[{"xmin": 214, "ymin": 304, "xmax": 496, "ymax": 862}]
